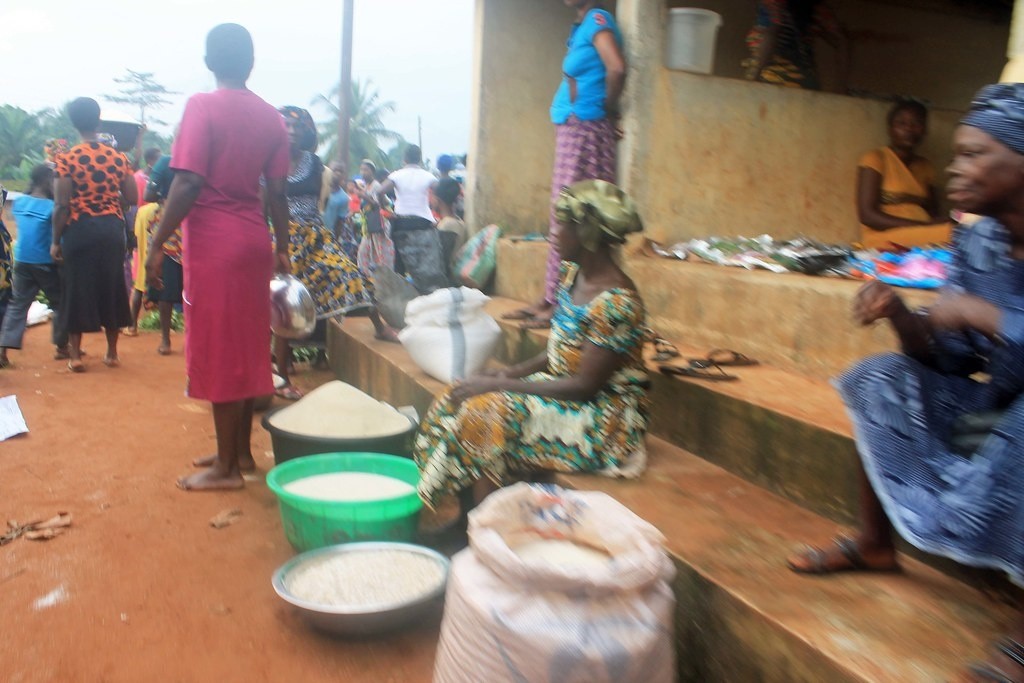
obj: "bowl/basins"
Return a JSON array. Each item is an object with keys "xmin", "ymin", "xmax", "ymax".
[
  {"xmin": 260, "ymin": 404, "xmax": 418, "ymax": 468},
  {"xmin": 272, "ymin": 541, "xmax": 452, "ymax": 639},
  {"xmin": 266, "ymin": 452, "xmax": 426, "ymax": 550},
  {"xmin": 266, "ymin": 271, "xmax": 316, "ymax": 340}
]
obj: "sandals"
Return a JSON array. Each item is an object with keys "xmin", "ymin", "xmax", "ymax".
[
  {"xmin": 55, "ymin": 346, "xmax": 86, "ymax": 358},
  {"xmin": 0, "ymin": 358, "xmax": 15, "ymax": 369}
]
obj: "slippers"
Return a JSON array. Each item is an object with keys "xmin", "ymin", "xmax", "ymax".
[
  {"xmin": 659, "ymin": 359, "xmax": 737, "ymax": 381},
  {"xmin": 274, "ymin": 386, "xmax": 304, "ymax": 400},
  {"xmin": 68, "ymin": 359, "xmax": 85, "ymax": 371},
  {"xmin": 690, "ymin": 349, "xmax": 752, "ymax": 368},
  {"xmin": 501, "ymin": 309, "xmax": 550, "ymax": 328},
  {"xmin": 123, "ymin": 326, "xmax": 137, "ymax": 336},
  {"xmin": 651, "ymin": 342, "xmax": 679, "ymax": 360},
  {"xmin": 104, "ymin": 354, "xmax": 120, "ymax": 367},
  {"xmin": 786, "ymin": 537, "xmax": 901, "ymax": 573},
  {"xmin": 375, "ymin": 327, "xmax": 401, "ymax": 343},
  {"xmin": 969, "ymin": 636, "xmax": 1024, "ymax": 683}
]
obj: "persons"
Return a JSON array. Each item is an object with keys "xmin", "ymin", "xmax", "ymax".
[
  {"xmin": 855, "ymin": 99, "xmax": 958, "ymax": 251},
  {"xmin": 50, "ymin": 97, "xmax": 137, "ymax": 371},
  {"xmin": 145, "ymin": 23, "xmax": 292, "ymax": 491},
  {"xmin": 785, "ymin": 82, "xmax": 1024, "ymax": 683},
  {"xmin": 414, "ymin": 178, "xmax": 653, "ymax": 535},
  {"xmin": 0, "ymin": 163, "xmax": 86, "ymax": 370},
  {"xmin": 260, "ymin": 105, "xmax": 402, "ymax": 401},
  {"xmin": 501, "ymin": 0, "xmax": 625, "ymax": 331},
  {"xmin": 44, "ymin": 126, "xmax": 467, "ymax": 360}
]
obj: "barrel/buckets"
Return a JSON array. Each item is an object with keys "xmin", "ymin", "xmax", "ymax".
[{"xmin": 665, "ymin": 6, "xmax": 723, "ymax": 75}]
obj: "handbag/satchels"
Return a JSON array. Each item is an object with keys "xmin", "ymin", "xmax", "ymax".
[{"xmin": 360, "ymin": 198, "xmax": 382, "ymax": 233}]
